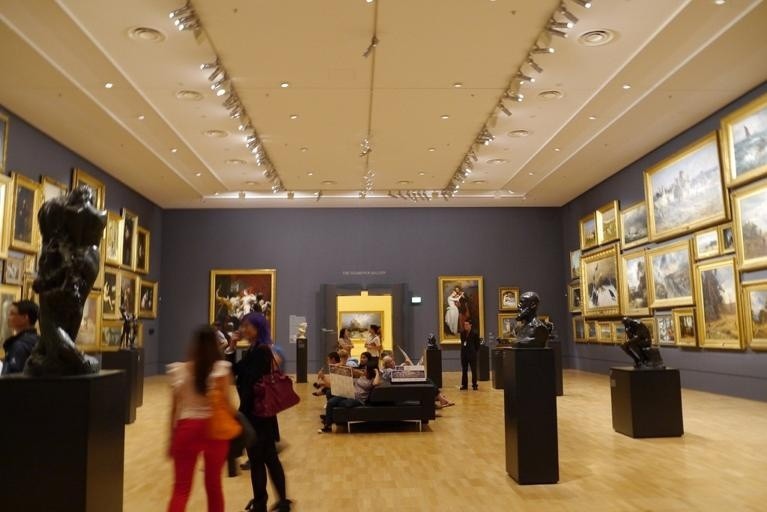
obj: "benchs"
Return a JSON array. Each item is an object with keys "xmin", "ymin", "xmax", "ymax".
[{"xmin": 332, "ymin": 383, "xmax": 436, "ymax": 433}]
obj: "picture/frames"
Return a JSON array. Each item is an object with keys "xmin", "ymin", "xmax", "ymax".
[
  {"xmin": 499, "ymin": 287, "xmax": 519, "ymax": 310},
  {"xmin": 539, "ymin": 314, "xmax": 550, "ymax": 321},
  {"xmin": 438, "ymin": 276, "xmax": 484, "ymax": 344},
  {"xmin": 209, "ymin": 269, "xmax": 276, "ymax": 348},
  {"xmin": 339, "ymin": 311, "xmax": 384, "ymax": 343},
  {"xmin": 498, "ymin": 314, "xmax": 518, "ymax": 336},
  {"xmin": 0, "ymin": 115, "xmax": 159, "ymax": 359}
]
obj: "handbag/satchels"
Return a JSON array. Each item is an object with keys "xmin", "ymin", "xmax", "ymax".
[
  {"xmin": 253, "ymin": 373, "xmax": 301, "ymax": 418},
  {"xmin": 208, "ymin": 395, "xmax": 243, "ymax": 441}
]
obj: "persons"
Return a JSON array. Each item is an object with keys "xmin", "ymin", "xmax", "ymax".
[
  {"xmin": 119, "ymin": 304, "xmax": 140, "ymax": 350},
  {"xmin": 165, "ymin": 312, "xmax": 292, "ymax": 512},
  {"xmin": 620, "ymin": 317, "xmax": 652, "ymax": 369},
  {"xmin": 312, "ymin": 325, "xmax": 455, "ymax": 433},
  {"xmin": 515, "ymin": 291, "xmax": 549, "ymax": 347},
  {"xmin": 0, "ymin": 299, "xmax": 40, "ymax": 375},
  {"xmin": 459, "ymin": 320, "xmax": 481, "ymax": 391},
  {"xmin": 215, "ymin": 279, "xmax": 272, "ymax": 324},
  {"xmin": 444, "ymin": 286, "xmax": 472, "ymax": 337}
]
